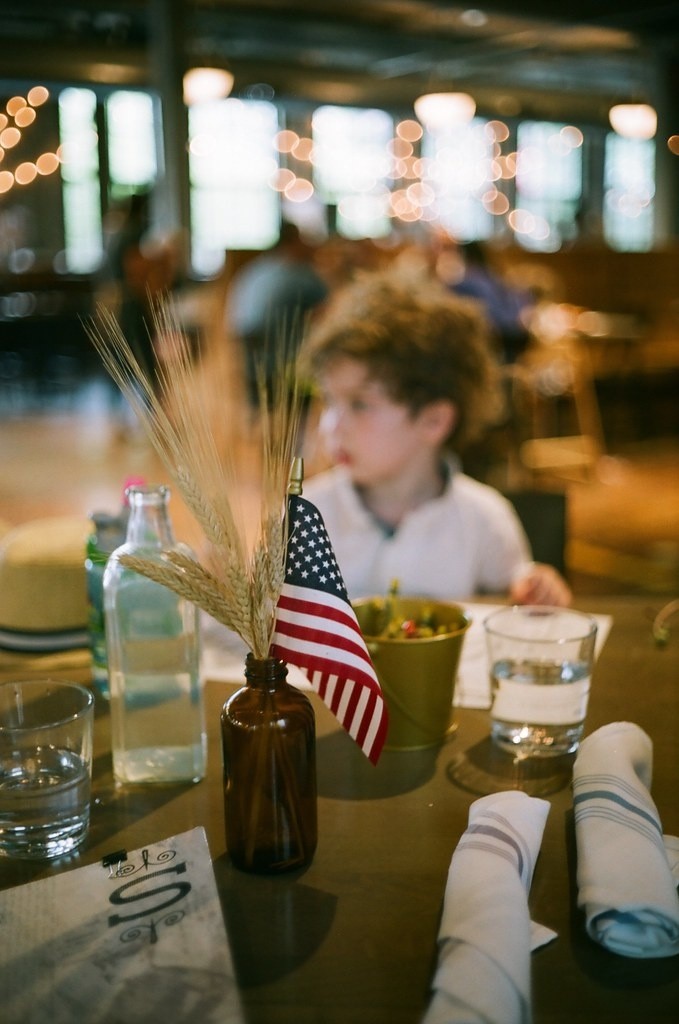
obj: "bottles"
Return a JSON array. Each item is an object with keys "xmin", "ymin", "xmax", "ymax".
[{"xmin": 84, "ymin": 478, "xmax": 208, "ymax": 785}]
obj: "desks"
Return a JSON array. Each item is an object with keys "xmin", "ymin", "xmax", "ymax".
[{"xmin": 0, "ymin": 590, "xmax": 679, "ymax": 1024}]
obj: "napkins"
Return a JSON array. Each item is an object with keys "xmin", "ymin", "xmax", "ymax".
[
  {"xmin": 572, "ymin": 723, "xmax": 679, "ymax": 957},
  {"xmin": 422, "ymin": 791, "xmax": 557, "ymax": 1024}
]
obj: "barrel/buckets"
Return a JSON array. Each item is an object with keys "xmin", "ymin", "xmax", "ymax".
[{"xmin": 351, "ymin": 597, "xmax": 475, "ymax": 752}]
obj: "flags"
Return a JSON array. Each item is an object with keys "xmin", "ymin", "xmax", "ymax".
[{"xmin": 268, "ymin": 495, "xmax": 389, "ymax": 767}]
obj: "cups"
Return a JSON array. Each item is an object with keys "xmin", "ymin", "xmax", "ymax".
[
  {"xmin": 482, "ymin": 603, "xmax": 598, "ymax": 758},
  {"xmin": 0, "ymin": 678, "xmax": 96, "ymax": 859}
]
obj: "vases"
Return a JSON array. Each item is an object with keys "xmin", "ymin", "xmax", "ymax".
[{"xmin": 220, "ymin": 650, "xmax": 317, "ymax": 872}]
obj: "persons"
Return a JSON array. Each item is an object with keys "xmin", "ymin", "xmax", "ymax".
[
  {"xmin": 297, "ymin": 273, "xmax": 573, "ymax": 607},
  {"xmin": 447, "ymin": 242, "xmax": 526, "ymax": 348},
  {"xmin": 230, "ymin": 223, "xmax": 330, "ymax": 337},
  {"xmin": 111, "ymin": 194, "xmax": 157, "ymax": 380}
]
